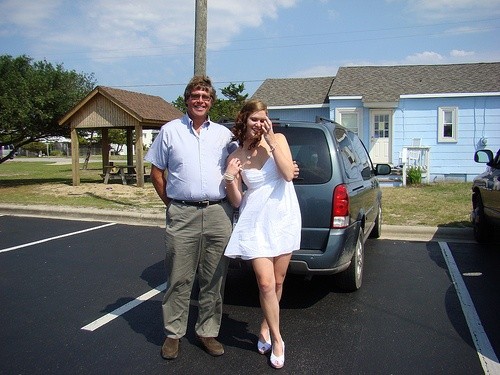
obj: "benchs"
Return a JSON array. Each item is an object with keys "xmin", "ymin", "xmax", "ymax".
[
  {"xmin": 123, "ymin": 174, "xmax": 151, "ymax": 185},
  {"xmin": 99, "ymin": 174, "xmax": 131, "ymax": 182}
]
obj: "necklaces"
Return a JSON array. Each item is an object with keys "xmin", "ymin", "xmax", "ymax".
[{"xmin": 245, "ymin": 155, "xmax": 253, "ymax": 159}]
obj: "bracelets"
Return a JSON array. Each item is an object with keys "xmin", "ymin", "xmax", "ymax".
[
  {"xmin": 269, "ymin": 143, "xmax": 279, "ymax": 152},
  {"xmin": 223, "ymin": 172, "xmax": 234, "ymax": 181}
]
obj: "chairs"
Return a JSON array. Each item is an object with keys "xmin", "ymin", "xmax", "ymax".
[{"xmin": 294, "ymin": 143, "xmax": 326, "ymax": 170}]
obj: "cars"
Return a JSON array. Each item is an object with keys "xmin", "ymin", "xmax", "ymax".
[{"xmin": 471, "ymin": 148, "xmax": 500, "ymax": 254}]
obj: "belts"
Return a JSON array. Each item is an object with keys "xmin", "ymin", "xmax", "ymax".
[{"xmin": 170, "ymin": 197, "xmax": 227, "ymax": 208}]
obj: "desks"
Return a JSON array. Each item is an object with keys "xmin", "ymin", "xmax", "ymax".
[{"xmin": 104, "ymin": 165, "xmax": 147, "ymax": 186}]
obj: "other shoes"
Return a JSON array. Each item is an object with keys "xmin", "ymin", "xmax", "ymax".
[
  {"xmin": 162, "ymin": 337, "xmax": 179, "ymax": 359},
  {"xmin": 197, "ymin": 336, "xmax": 224, "ymax": 355}
]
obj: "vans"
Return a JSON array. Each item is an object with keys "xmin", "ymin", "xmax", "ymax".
[{"xmin": 218, "ymin": 116, "xmax": 392, "ymax": 294}]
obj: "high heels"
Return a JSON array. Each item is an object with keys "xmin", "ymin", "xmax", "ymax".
[
  {"xmin": 257, "ymin": 333, "xmax": 271, "ymax": 354},
  {"xmin": 270, "ymin": 338, "xmax": 285, "ymax": 368}
]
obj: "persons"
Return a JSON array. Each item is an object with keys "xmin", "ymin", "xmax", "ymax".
[
  {"xmin": 226, "ymin": 101, "xmax": 302, "ymax": 368},
  {"xmin": 145, "ymin": 76, "xmax": 299, "ymax": 358}
]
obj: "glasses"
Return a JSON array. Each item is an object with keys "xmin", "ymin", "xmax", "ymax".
[{"xmin": 189, "ymin": 94, "xmax": 212, "ymax": 101}]
